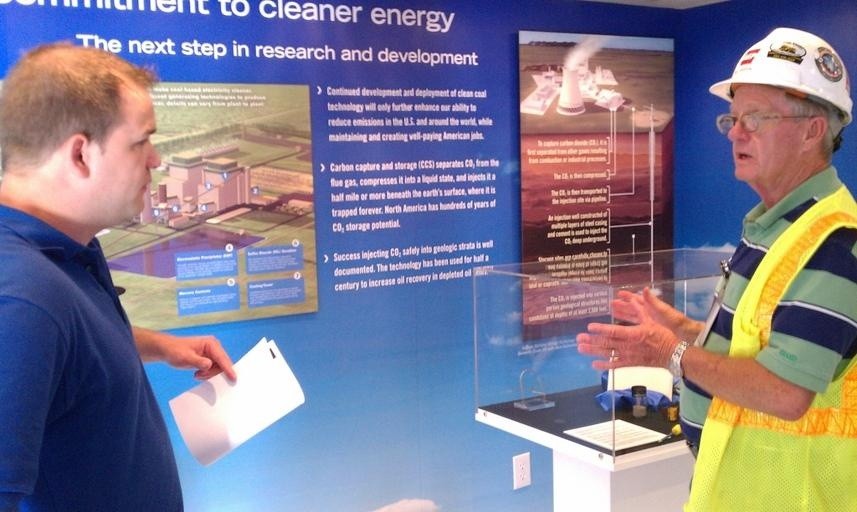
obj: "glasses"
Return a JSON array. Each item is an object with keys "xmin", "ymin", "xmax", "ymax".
[{"xmin": 715, "ymin": 113, "xmax": 819, "ymax": 136}]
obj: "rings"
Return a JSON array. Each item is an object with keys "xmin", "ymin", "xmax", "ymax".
[{"xmin": 609, "ymin": 347, "xmax": 620, "ymax": 362}]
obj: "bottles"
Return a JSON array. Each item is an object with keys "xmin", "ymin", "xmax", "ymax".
[
  {"xmin": 632, "ymin": 386, "xmax": 649, "ymax": 419},
  {"xmin": 666, "ymin": 404, "xmax": 679, "ymax": 422}
]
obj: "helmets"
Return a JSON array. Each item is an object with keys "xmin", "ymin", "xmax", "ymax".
[{"xmin": 707, "ymin": 26, "xmax": 855, "ymax": 125}]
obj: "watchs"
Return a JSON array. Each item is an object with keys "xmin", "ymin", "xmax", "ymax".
[{"xmin": 668, "ymin": 340, "xmax": 690, "ymax": 379}]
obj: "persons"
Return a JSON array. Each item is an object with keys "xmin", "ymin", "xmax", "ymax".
[
  {"xmin": 0, "ymin": 41, "xmax": 237, "ymax": 510},
  {"xmin": 575, "ymin": 26, "xmax": 856, "ymax": 511}
]
